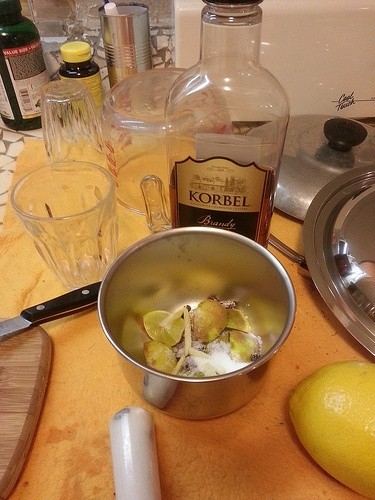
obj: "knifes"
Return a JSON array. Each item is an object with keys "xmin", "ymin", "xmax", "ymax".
[{"xmin": 0, "ymin": 280, "xmax": 102, "ymax": 342}]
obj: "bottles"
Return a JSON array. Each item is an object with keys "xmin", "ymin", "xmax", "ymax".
[
  {"xmin": 57, "ymin": 39, "xmax": 106, "ymax": 120},
  {"xmin": 0, "ymin": 0, "xmax": 48, "ymax": 130},
  {"xmin": 163, "ymin": 0, "xmax": 287, "ymax": 249}
]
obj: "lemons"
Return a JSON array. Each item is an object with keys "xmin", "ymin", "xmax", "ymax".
[
  {"xmin": 288, "ymin": 360, "xmax": 375, "ymax": 499},
  {"xmin": 138, "ymin": 298, "xmax": 261, "ymax": 373}
]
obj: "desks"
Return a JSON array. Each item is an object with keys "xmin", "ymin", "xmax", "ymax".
[{"xmin": 1, "ymin": 136, "xmax": 374, "ymax": 500}]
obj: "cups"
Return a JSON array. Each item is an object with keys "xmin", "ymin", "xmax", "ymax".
[
  {"xmin": 97, "ymin": 3, "xmax": 152, "ymax": 90},
  {"xmin": 10, "ymin": 161, "xmax": 117, "ymax": 291},
  {"xmin": 40, "ymin": 80, "xmax": 106, "ymax": 174},
  {"xmin": 104, "ymin": 66, "xmax": 232, "ymax": 233}
]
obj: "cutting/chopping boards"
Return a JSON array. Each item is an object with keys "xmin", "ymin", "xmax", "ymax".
[{"xmin": 1, "ymin": 318, "xmax": 54, "ymax": 500}]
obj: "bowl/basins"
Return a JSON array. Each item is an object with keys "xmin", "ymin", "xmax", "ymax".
[{"xmin": 96, "ymin": 225, "xmax": 298, "ymax": 420}]
